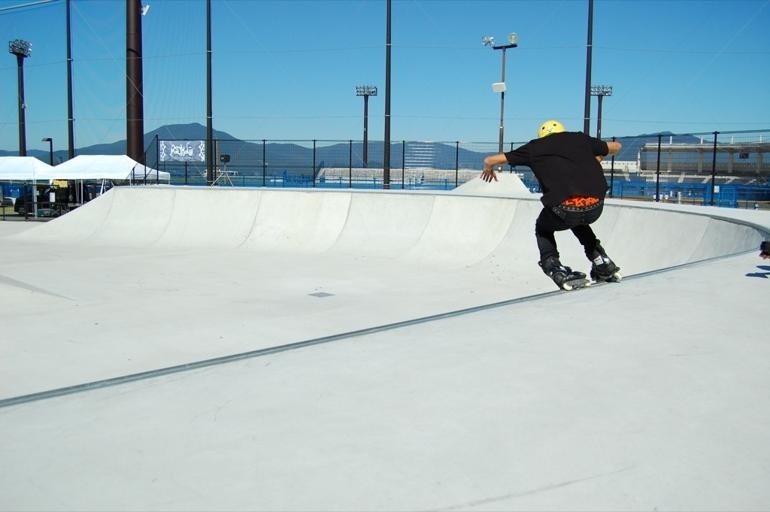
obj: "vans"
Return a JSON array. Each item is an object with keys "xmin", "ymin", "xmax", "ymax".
[{"xmin": 15, "ymin": 184, "xmax": 90, "ymax": 215}]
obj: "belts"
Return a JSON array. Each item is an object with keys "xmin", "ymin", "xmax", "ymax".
[{"xmin": 551, "ymin": 200, "xmax": 603, "ymax": 219}]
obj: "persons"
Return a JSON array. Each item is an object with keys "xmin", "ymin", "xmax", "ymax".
[{"xmin": 480, "ymin": 119, "xmax": 622, "ymax": 286}]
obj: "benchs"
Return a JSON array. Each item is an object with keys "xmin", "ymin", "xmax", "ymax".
[{"xmin": 25, "ymin": 202, "xmax": 62, "ymax": 222}]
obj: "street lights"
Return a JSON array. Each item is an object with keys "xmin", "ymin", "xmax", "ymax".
[
  {"xmin": 9, "ymin": 38, "xmax": 32, "ymax": 156},
  {"xmin": 41, "ymin": 138, "xmax": 52, "ymax": 165},
  {"xmin": 356, "ymin": 86, "xmax": 377, "ymax": 166},
  {"xmin": 591, "ymin": 86, "xmax": 612, "ymax": 141},
  {"xmin": 483, "ymin": 32, "xmax": 517, "ymax": 173}
]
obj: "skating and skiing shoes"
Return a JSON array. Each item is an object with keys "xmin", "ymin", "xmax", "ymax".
[
  {"xmin": 586, "ymin": 240, "xmax": 622, "ymax": 282},
  {"xmin": 537, "ymin": 254, "xmax": 592, "ymax": 291}
]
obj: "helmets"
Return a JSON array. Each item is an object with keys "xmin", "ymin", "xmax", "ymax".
[{"xmin": 538, "ymin": 121, "xmax": 565, "ymax": 138}]
